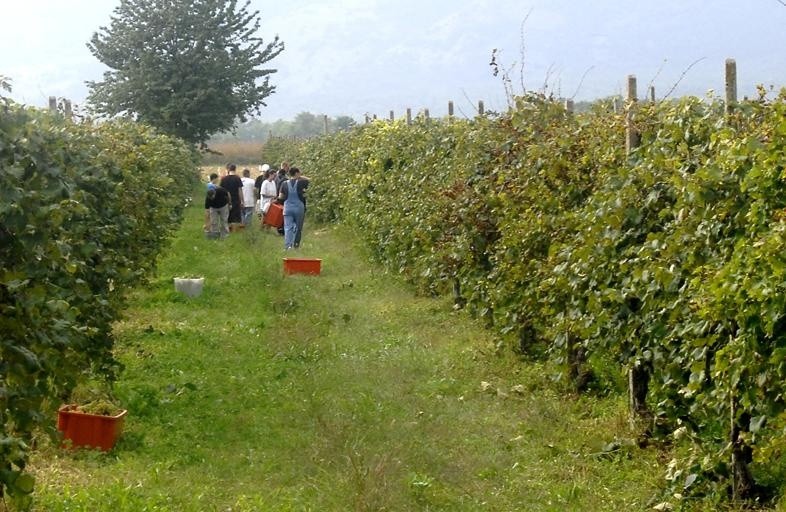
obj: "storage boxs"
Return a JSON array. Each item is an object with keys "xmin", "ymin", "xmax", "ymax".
[
  {"xmin": 203, "ymin": 228, "xmax": 221, "ymax": 241},
  {"xmin": 262, "ymin": 201, "xmax": 284, "ymax": 229},
  {"xmin": 55, "ymin": 403, "xmax": 128, "ymax": 456},
  {"xmin": 172, "ymin": 276, "xmax": 206, "ymax": 299},
  {"xmin": 228, "ymin": 223, "xmax": 246, "ymax": 233},
  {"xmin": 281, "ymin": 257, "xmax": 323, "ymax": 277}
]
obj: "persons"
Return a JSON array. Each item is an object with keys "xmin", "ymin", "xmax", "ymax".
[{"xmin": 202, "ymin": 160, "xmax": 312, "ymax": 250}]
